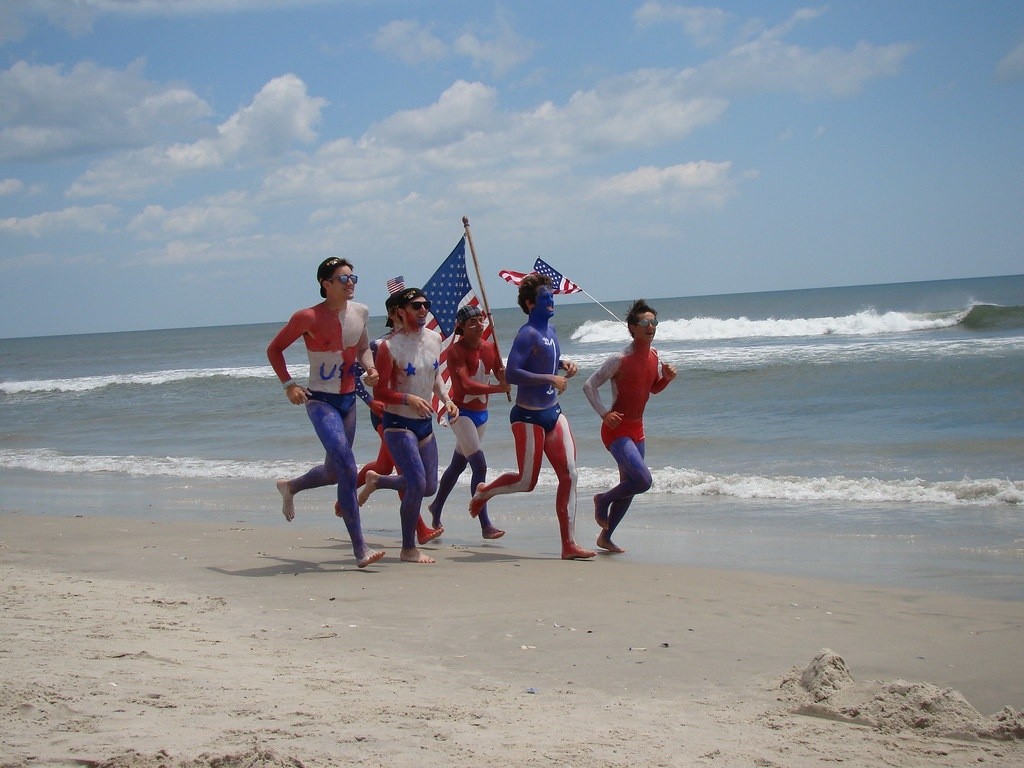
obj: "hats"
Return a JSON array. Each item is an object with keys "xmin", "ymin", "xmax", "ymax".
[
  {"xmin": 455, "ymin": 305, "xmax": 483, "ymax": 335},
  {"xmin": 317, "ymin": 257, "xmax": 342, "ymax": 298},
  {"xmin": 385, "ymin": 293, "xmax": 399, "ymax": 327},
  {"xmin": 397, "ymin": 288, "xmax": 423, "ymax": 323}
]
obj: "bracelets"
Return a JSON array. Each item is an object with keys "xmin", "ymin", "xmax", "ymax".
[
  {"xmin": 445, "ymin": 400, "xmax": 454, "ymax": 405},
  {"xmin": 402, "ymin": 392, "xmax": 409, "ymax": 405},
  {"xmin": 282, "ymin": 379, "xmax": 295, "ymax": 391}
]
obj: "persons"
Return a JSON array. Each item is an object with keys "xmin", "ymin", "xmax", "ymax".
[
  {"xmin": 468, "ymin": 273, "xmax": 597, "ymax": 560},
  {"xmin": 335, "ymin": 290, "xmax": 443, "ymax": 545},
  {"xmin": 358, "ymin": 288, "xmax": 460, "ymax": 563},
  {"xmin": 582, "ymin": 300, "xmax": 677, "ymax": 553},
  {"xmin": 267, "ymin": 256, "xmax": 386, "ymax": 568},
  {"xmin": 427, "ymin": 305, "xmax": 511, "ymax": 539}
]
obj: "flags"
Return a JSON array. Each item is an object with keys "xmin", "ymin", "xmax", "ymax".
[
  {"xmin": 498, "ymin": 257, "xmax": 582, "ymax": 295},
  {"xmin": 386, "ymin": 276, "xmax": 404, "ymax": 294},
  {"xmin": 420, "ymin": 236, "xmax": 494, "ymax": 425}
]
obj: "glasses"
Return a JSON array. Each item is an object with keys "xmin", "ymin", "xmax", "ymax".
[
  {"xmin": 635, "ymin": 320, "xmax": 658, "ymax": 328},
  {"xmin": 402, "ymin": 301, "xmax": 430, "ymax": 310},
  {"xmin": 328, "ymin": 274, "xmax": 357, "ymax": 284}
]
